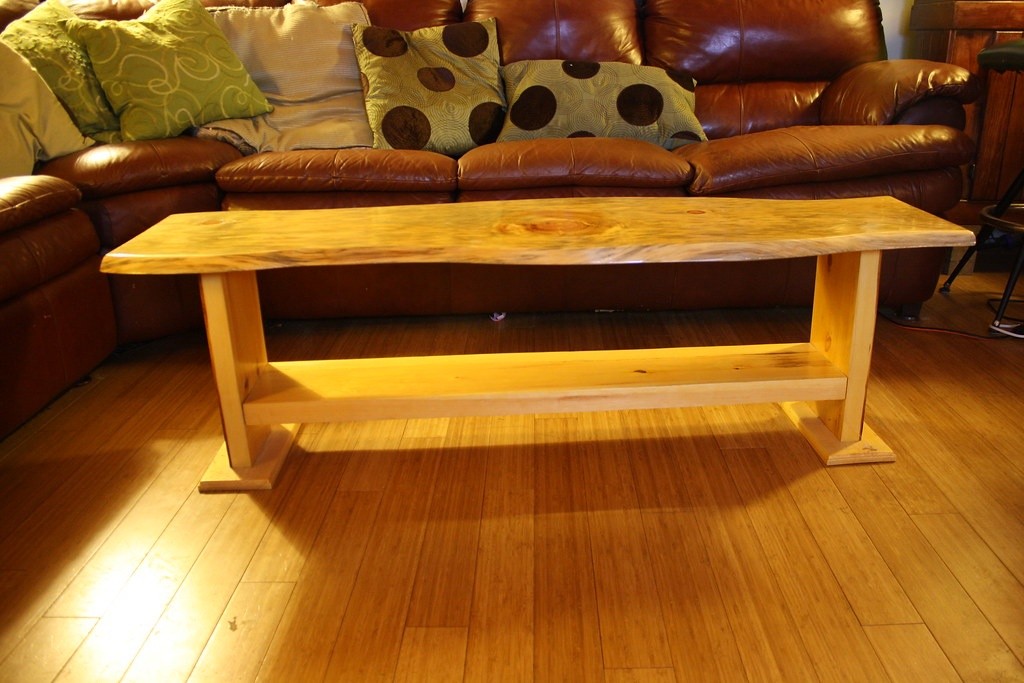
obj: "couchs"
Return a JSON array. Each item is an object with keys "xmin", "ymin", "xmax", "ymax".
[{"xmin": 2, "ymin": 0, "xmax": 981, "ymax": 438}]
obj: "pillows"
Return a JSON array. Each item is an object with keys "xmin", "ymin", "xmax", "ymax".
[
  {"xmin": 0, "ymin": 0, "xmax": 122, "ymax": 144},
  {"xmin": 56, "ymin": 0, "xmax": 273, "ymax": 143},
  {"xmin": 494, "ymin": 61, "xmax": 709, "ymax": 145},
  {"xmin": 349, "ymin": 18, "xmax": 507, "ymax": 156},
  {"xmin": 192, "ymin": 1, "xmax": 372, "ymax": 154},
  {"xmin": 0, "ymin": 43, "xmax": 96, "ymax": 180}
]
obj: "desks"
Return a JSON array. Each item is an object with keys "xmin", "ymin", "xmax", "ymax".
[{"xmin": 100, "ymin": 195, "xmax": 973, "ymax": 493}]
